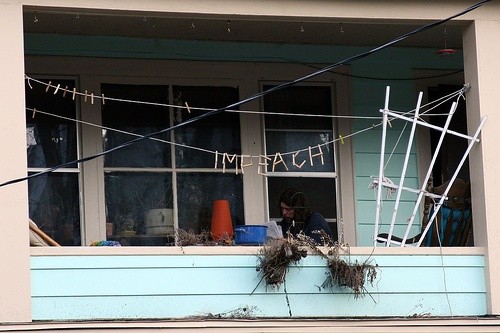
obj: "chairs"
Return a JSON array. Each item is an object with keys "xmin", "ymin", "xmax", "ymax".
[{"xmin": 376, "ymin": 176, "xmax": 475, "ymax": 246}]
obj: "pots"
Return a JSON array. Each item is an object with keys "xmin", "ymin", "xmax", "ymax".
[{"xmin": 232, "ymin": 224, "xmax": 268, "ymax": 246}]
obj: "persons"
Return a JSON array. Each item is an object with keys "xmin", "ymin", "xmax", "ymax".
[{"xmin": 277, "ymin": 188, "xmax": 334, "ymax": 247}]
